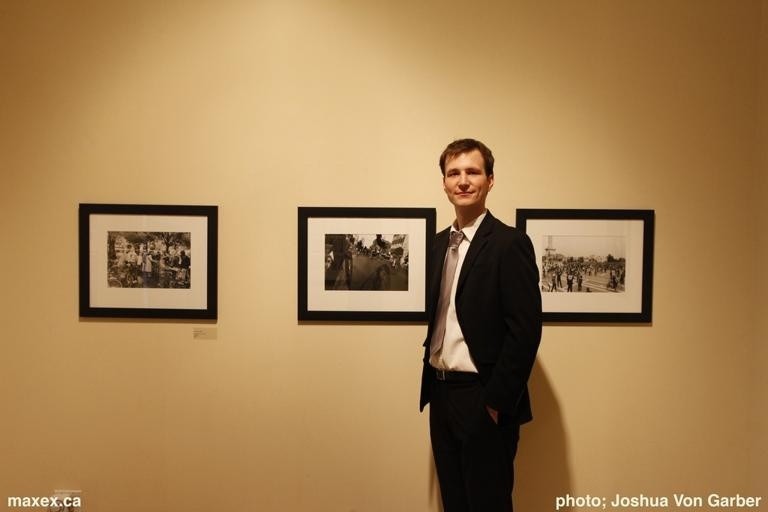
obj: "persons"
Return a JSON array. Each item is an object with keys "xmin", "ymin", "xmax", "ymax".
[
  {"xmin": 541, "ymin": 259, "xmax": 625, "ymax": 292},
  {"xmin": 419, "ymin": 138, "xmax": 542, "ymax": 512},
  {"xmin": 107, "ymin": 244, "xmax": 190, "ymax": 288}
]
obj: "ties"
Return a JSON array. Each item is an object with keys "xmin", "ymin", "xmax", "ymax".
[{"xmin": 428, "ymin": 230, "xmax": 466, "ymax": 372}]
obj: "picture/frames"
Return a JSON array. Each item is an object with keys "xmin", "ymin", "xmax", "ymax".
[
  {"xmin": 296, "ymin": 207, "xmax": 439, "ymax": 322},
  {"xmin": 77, "ymin": 202, "xmax": 220, "ymax": 321},
  {"xmin": 515, "ymin": 208, "xmax": 657, "ymax": 324}
]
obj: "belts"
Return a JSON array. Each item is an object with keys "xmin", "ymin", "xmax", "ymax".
[{"xmin": 427, "ymin": 365, "xmax": 480, "ymax": 384}]
